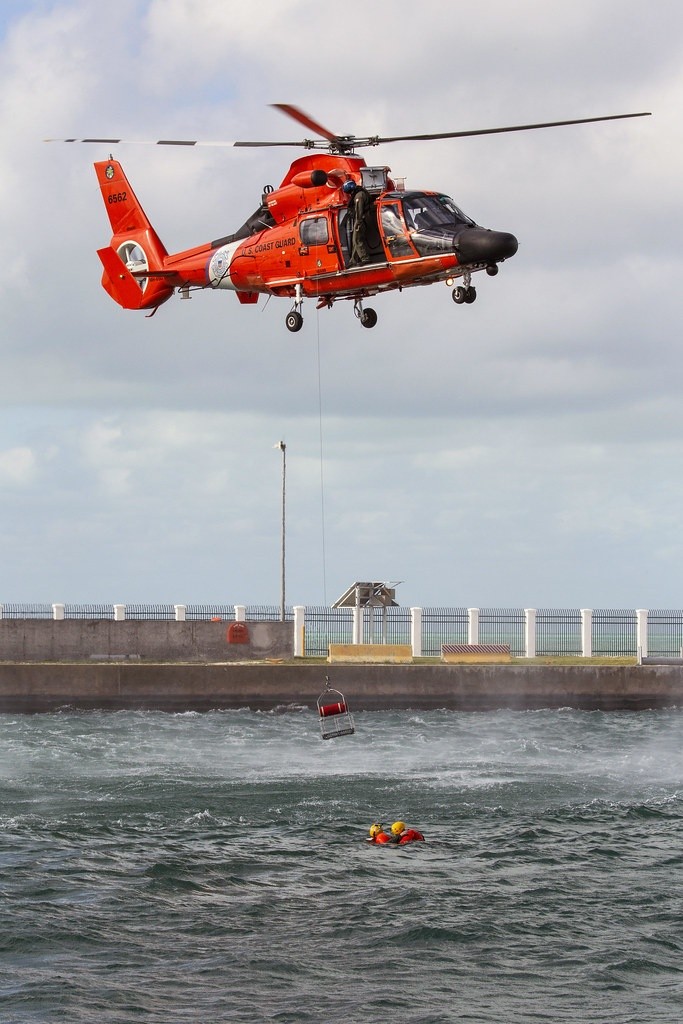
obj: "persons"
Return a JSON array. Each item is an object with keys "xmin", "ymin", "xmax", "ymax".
[
  {"xmin": 391, "ymin": 822, "xmax": 425, "ymax": 844},
  {"xmin": 342, "ymin": 181, "xmax": 371, "ymax": 265},
  {"xmin": 370, "ymin": 824, "xmax": 407, "ymax": 843}
]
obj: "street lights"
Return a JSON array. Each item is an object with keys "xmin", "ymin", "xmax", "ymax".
[{"xmin": 277, "ymin": 441, "xmax": 286, "ymax": 622}]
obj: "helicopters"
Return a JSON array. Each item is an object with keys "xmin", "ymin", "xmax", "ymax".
[{"xmin": 41, "ymin": 104, "xmax": 652, "ymax": 331}]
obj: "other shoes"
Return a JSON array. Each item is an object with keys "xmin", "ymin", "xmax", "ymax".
[
  {"xmin": 345, "ymin": 262, "xmax": 356, "ymax": 269},
  {"xmin": 357, "ymin": 261, "xmax": 373, "ymax": 267}
]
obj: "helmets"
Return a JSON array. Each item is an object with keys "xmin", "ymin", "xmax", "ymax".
[
  {"xmin": 391, "ymin": 822, "xmax": 406, "ymax": 835},
  {"xmin": 370, "ymin": 824, "xmax": 383, "ymax": 837},
  {"xmin": 343, "ymin": 180, "xmax": 356, "ymax": 194}
]
